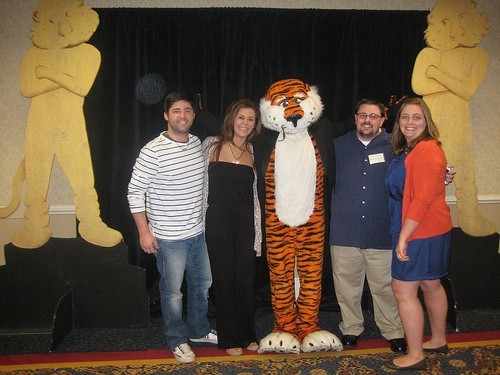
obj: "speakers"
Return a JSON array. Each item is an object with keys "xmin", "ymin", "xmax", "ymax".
[{"xmin": 71, "ymin": 257, "xmax": 152, "ymax": 329}]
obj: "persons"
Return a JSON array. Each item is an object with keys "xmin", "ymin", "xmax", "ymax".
[
  {"xmin": 384, "ymin": 96, "xmax": 453, "ymax": 372},
  {"xmin": 203, "ymin": 99, "xmax": 263, "ymax": 357},
  {"xmin": 128, "ymin": 92, "xmax": 217, "ymax": 363},
  {"xmin": 329, "ymin": 100, "xmax": 456, "ymax": 353}
]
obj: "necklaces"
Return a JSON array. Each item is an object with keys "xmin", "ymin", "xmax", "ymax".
[
  {"xmin": 229, "ymin": 141, "xmax": 245, "ymax": 165},
  {"xmin": 171, "ymin": 134, "xmax": 190, "ymax": 151}
]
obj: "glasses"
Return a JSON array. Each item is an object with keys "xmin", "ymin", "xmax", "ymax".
[{"xmin": 356, "ymin": 112, "xmax": 382, "ymax": 119}]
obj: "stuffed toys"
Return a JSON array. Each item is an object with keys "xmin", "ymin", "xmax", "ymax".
[{"xmin": 258, "ymin": 79, "xmax": 343, "ymax": 354}]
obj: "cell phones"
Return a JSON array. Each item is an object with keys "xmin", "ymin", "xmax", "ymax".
[{"xmin": 444, "ymin": 167, "xmax": 452, "ymax": 185}]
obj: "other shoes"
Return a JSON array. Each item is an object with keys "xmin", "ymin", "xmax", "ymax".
[
  {"xmin": 226, "ymin": 347, "xmax": 243, "ymax": 356},
  {"xmin": 246, "ymin": 341, "xmax": 259, "ymax": 351},
  {"xmin": 421, "ymin": 345, "xmax": 448, "ymax": 356},
  {"xmin": 384, "ymin": 355, "xmax": 426, "ymax": 370}
]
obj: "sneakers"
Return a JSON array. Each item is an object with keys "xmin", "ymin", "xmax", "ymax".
[
  {"xmin": 189, "ymin": 332, "xmax": 218, "ymax": 346},
  {"xmin": 389, "ymin": 338, "xmax": 408, "ymax": 353},
  {"xmin": 172, "ymin": 340, "xmax": 195, "ymax": 363},
  {"xmin": 341, "ymin": 334, "xmax": 358, "ymax": 349}
]
